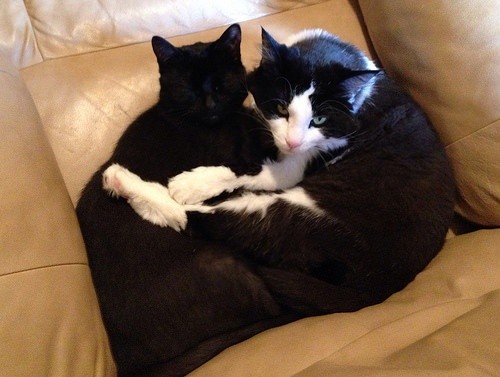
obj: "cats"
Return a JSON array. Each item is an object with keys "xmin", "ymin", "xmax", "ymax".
[
  {"xmin": 75, "ymin": 22, "xmax": 278, "ymax": 369},
  {"xmin": 102, "ymin": 24, "xmax": 453, "ymax": 314}
]
